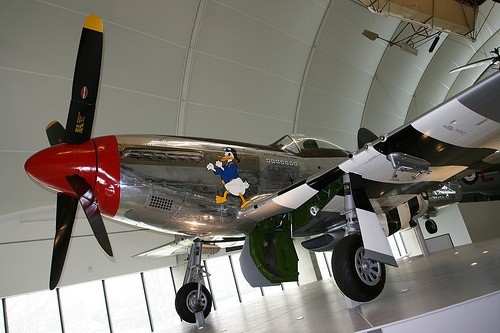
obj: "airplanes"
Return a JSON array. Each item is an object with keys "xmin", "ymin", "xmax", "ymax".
[{"xmin": 24, "ymin": 14, "xmax": 499, "ymax": 323}]
